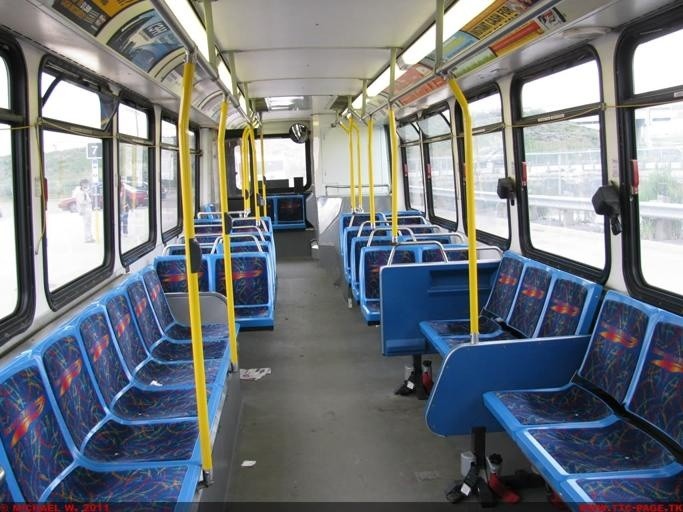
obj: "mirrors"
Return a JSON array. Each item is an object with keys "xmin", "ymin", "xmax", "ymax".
[{"xmin": 289, "ymin": 123, "xmax": 308, "ymax": 143}]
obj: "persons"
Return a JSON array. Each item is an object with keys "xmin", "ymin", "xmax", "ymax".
[
  {"xmin": 120, "ymin": 174, "xmax": 130, "ymax": 237},
  {"xmin": 77, "ymin": 178, "xmax": 97, "ymax": 243}
]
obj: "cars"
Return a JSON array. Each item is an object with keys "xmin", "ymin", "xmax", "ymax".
[{"xmin": 59, "ymin": 178, "xmax": 169, "ymax": 213}]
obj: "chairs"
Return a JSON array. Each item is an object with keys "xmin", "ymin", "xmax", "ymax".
[
  {"xmin": 339, "ymin": 211, "xmax": 682, "ymax": 512},
  {"xmin": 0, "ymin": 215, "xmax": 277, "ymax": 511}
]
